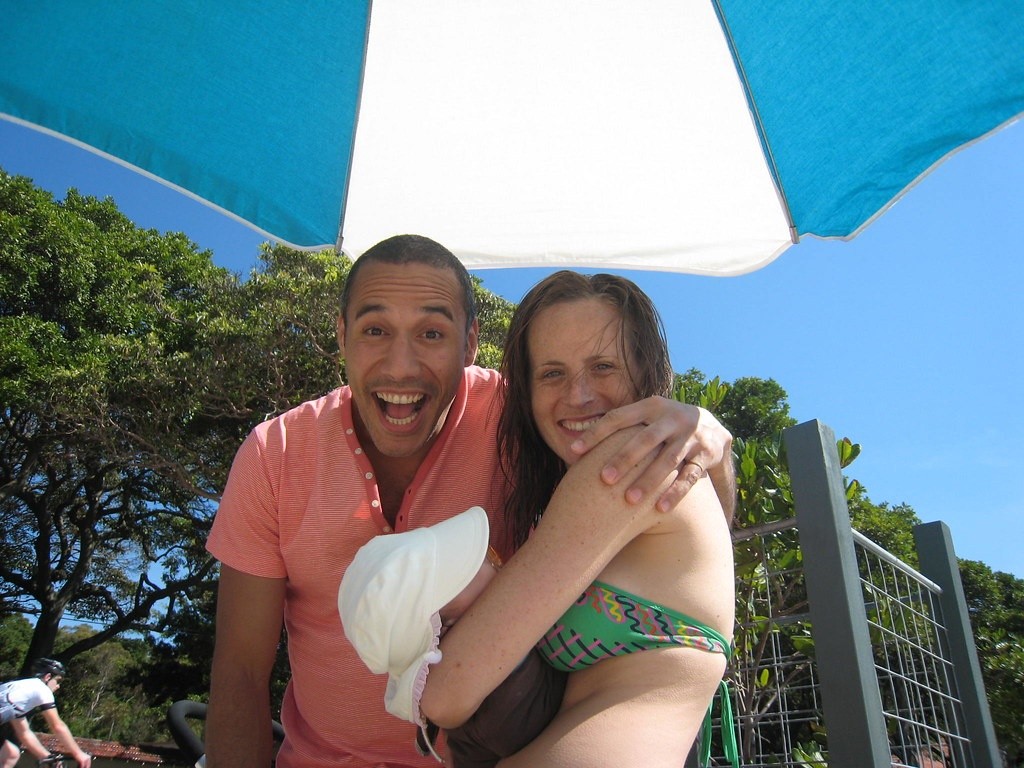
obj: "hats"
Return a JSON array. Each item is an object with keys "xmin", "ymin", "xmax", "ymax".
[{"xmin": 337, "ymin": 505, "xmax": 490, "ymax": 764}]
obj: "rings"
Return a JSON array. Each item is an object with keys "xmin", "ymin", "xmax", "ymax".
[{"xmin": 686, "ymin": 460, "xmax": 703, "ymax": 474}]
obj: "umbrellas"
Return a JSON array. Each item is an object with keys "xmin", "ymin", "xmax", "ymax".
[{"xmin": 1, "ymin": 1, "xmax": 1024, "ymax": 277}]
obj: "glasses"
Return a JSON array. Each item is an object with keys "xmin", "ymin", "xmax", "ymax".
[{"xmin": 53, "ymin": 678, "xmax": 63, "ymax": 684}]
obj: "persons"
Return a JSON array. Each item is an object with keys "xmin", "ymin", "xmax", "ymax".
[
  {"xmin": 204, "ymin": 235, "xmax": 736, "ymax": 768},
  {"xmin": 0, "ymin": 657, "xmax": 91, "ymax": 768},
  {"xmin": 418, "ymin": 268, "xmax": 735, "ymax": 768},
  {"xmin": 338, "ymin": 507, "xmax": 569, "ymax": 768}
]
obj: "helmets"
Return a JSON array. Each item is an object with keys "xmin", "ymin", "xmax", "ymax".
[{"xmin": 30, "ymin": 657, "xmax": 66, "ymax": 678}]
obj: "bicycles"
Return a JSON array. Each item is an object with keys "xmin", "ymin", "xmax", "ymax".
[{"xmin": 37, "ymin": 751, "xmax": 96, "ymax": 768}]
